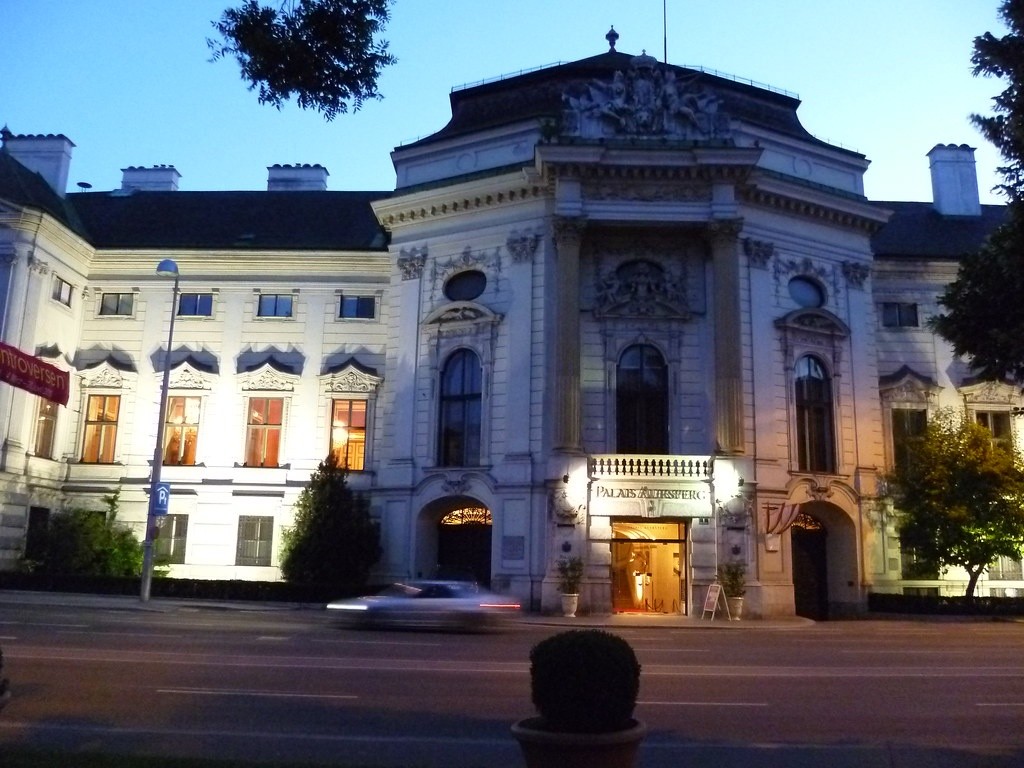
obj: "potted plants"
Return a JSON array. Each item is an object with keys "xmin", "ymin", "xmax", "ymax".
[
  {"xmin": 554, "ymin": 556, "xmax": 584, "ymax": 617},
  {"xmin": 510, "ymin": 630, "xmax": 650, "ymax": 768},
  {"xmin": 718, "ymin": 558, "xmax": 747, "ymax": 622}
]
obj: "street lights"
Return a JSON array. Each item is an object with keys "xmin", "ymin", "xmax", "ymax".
[{"xmin": 139, "ymin": 258, "xmax": 180, "ymax": 603}]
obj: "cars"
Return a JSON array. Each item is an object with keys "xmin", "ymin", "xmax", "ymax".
[{"xmin": 325, "ymin": 579, "xmax": 521, "ymax": 634}]
